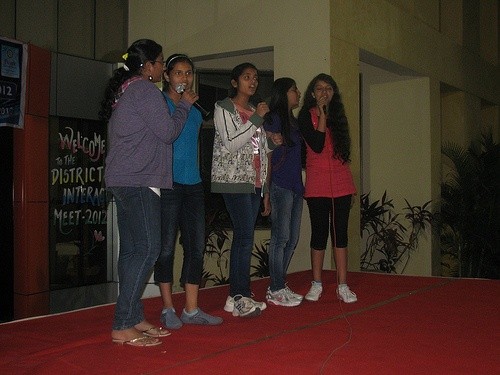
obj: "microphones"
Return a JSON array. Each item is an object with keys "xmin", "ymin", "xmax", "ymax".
[
  {"xmin": 321, "ymin": 95, "xmax": 328, "ymax": 114},
  {"xmin": 174, "ymin": 83, "xmax": 211, "ymax": 116},
  {"xmin": 253, "ymin": 94, "xmax": 272, "ymax": 124}
]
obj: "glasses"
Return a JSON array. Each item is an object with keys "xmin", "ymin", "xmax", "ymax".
[{"xmin": 144, "ymin": 60, "xmax": 166, "ymax": 68}]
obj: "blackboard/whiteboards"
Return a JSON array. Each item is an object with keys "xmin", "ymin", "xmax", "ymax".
[
  {"xmin": 47, "ymin": 114, "xmax": 109, "ymax": 292},
  {"xmin": 196, "ymin": 68, "xmax": 274, "ymax": 230}
]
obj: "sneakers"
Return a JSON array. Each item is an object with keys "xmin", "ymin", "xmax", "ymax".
[
  {"xmin": 160, "ymin": 309, "xmax": 182, "ymax": 329},
  {"xmin": 181, "ymin": 308, "xmax": 224, "ymax": 325},
  {"xmin": 266, "ymin": 286, "xmax": 303, "ymax": 307},
  {"xmin": 336, "ymin": 286, "xmax": 358, "ymax": 303},
  {"xmin": 232, "ymin": 295, "xmax": 260, "ymax": 318},
  {"xmin": 305, "ymin": 281, "xmax": 323, "ymax": 301},
  {"xmin": 224, "ymin": 295, "xmax": 266, "ymax": 313}
]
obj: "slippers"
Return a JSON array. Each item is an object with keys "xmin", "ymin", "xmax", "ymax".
[
  {"xmin": 111, "ymin": 330, "xmax": 161, "ymax": 347},
  {"xmin": 143, "ymin": 326, "xmax": 171, "ymax": 337}
]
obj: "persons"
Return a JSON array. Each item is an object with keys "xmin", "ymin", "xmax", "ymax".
[
  {"xmin": 153, "ymin": 54, "xmax": 222, "ymax": 329},
  {"xmin": 263, "ymin": 77, "xmax": 305, "ymax": 306},
  {"xmin": 298, "ymin": 73, "xmax": 357, "ymax": 303},
  {"xmin": 98, "ymin": 38, "xmax": 199, "ymax": 347},
  {"xmin": 210, "ymin": 63, "xmax": 282, "ymax": 317}
]
